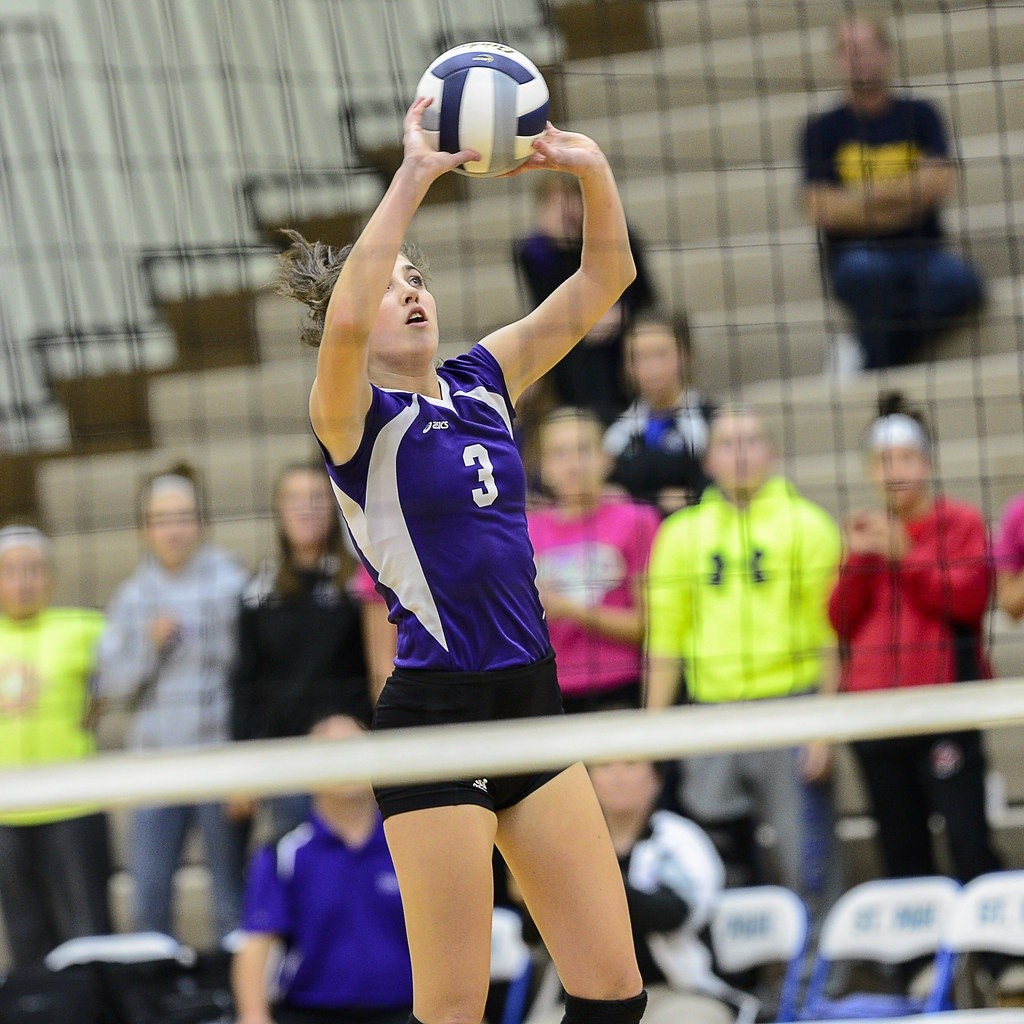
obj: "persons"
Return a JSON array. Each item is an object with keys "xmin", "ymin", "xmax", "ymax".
[
  {"xmin": 794, "ymin": 10, "xmax": 992, "ymax": 373},
  {"xmin": 306, "ymin": 94, "xmax": 648, "ymax": 1023},
  {"xmin": 1, "ymin": 163, "xmax": 1023, "ymax": 1024}
]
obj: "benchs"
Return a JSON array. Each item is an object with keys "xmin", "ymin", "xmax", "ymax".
[{"xmin": 0, "ymin": 0, "xmax": 1024, "ymax": 944}]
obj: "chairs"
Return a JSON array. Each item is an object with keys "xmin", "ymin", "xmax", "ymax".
[
  {"xmin": 45, "ymin": 933, "xmax": 180, "ymax": 970},
  {"xmin": 490, "ymin": 908, "xmax": 533, "ymax": 1024},
  {"xmin": 711, "ymin": 885, "xmax": 809, "ymax": 1024},
  {"xmin": 795, "ymin": 876, "xmax": 961, "ymax": 1024},
  {"xmin": 924, "ymin": 867, "xmax": 1024, "ymax": 1024}
]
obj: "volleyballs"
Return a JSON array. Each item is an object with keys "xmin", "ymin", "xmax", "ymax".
[{"xmin": 413, "ymin": 40, "xmax": 550, "ymax": 178}]
